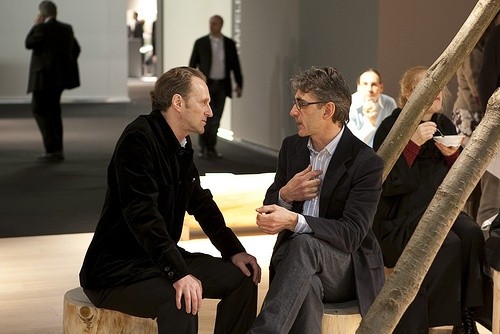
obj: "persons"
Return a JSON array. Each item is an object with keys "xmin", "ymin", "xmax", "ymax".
[
  {"xmin": 373, "ymin": 65, "xmax": 486, "ymax": 334},
  {"xmin": 79, "ymin": 67, "xmax": 261, "ymax": 334},
  {"xmin": 451, "ymin": 10, "xmax": 500, "ymax": 271},
  {"xmin": 189, "ymin": 15, "xmax": 243, "ymax": 159},
  {"xmin": 345, "ymin": 69, "xmax": 397, "ymax": 148},
  {"xmin": 126, "ymin": 12, "xmax": 157, "ymax": 65},
  {"xmin": 248, "ymin": 66, "xmax": 386, "ymax": 334},
  {"xmin": 25, "ymin": 0, "xmax": 81, "ymax": 162}
]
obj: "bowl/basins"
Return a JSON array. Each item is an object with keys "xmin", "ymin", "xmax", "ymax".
[{"xmin": 433, "ymin": 135, "xmax": 465, "ymax": 147}]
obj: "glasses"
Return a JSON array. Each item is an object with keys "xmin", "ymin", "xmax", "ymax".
[{"xmin": 292, "ymin": 100, "xmax": 328, "ymax": 110}]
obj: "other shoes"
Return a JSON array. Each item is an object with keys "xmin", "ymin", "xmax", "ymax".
[
  {"xmin": 38, "ymin": 153, "xmax": 64, "ymax": 161},
  {"xmin": 209, "ymin": 151, "xmax": 223, "ymax": 158},
  {"xmin": 198, "ymin": 147, "xmax": 204, "ymax": 156}
]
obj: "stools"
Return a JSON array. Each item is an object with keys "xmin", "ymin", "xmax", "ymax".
[
  {"xmin": 322, "ymin": 299, "xmax": 362, "ymax": 334},
  {"xmin": 63, "ymin": 287, "xmax": 158, "ymax": 334}
]
